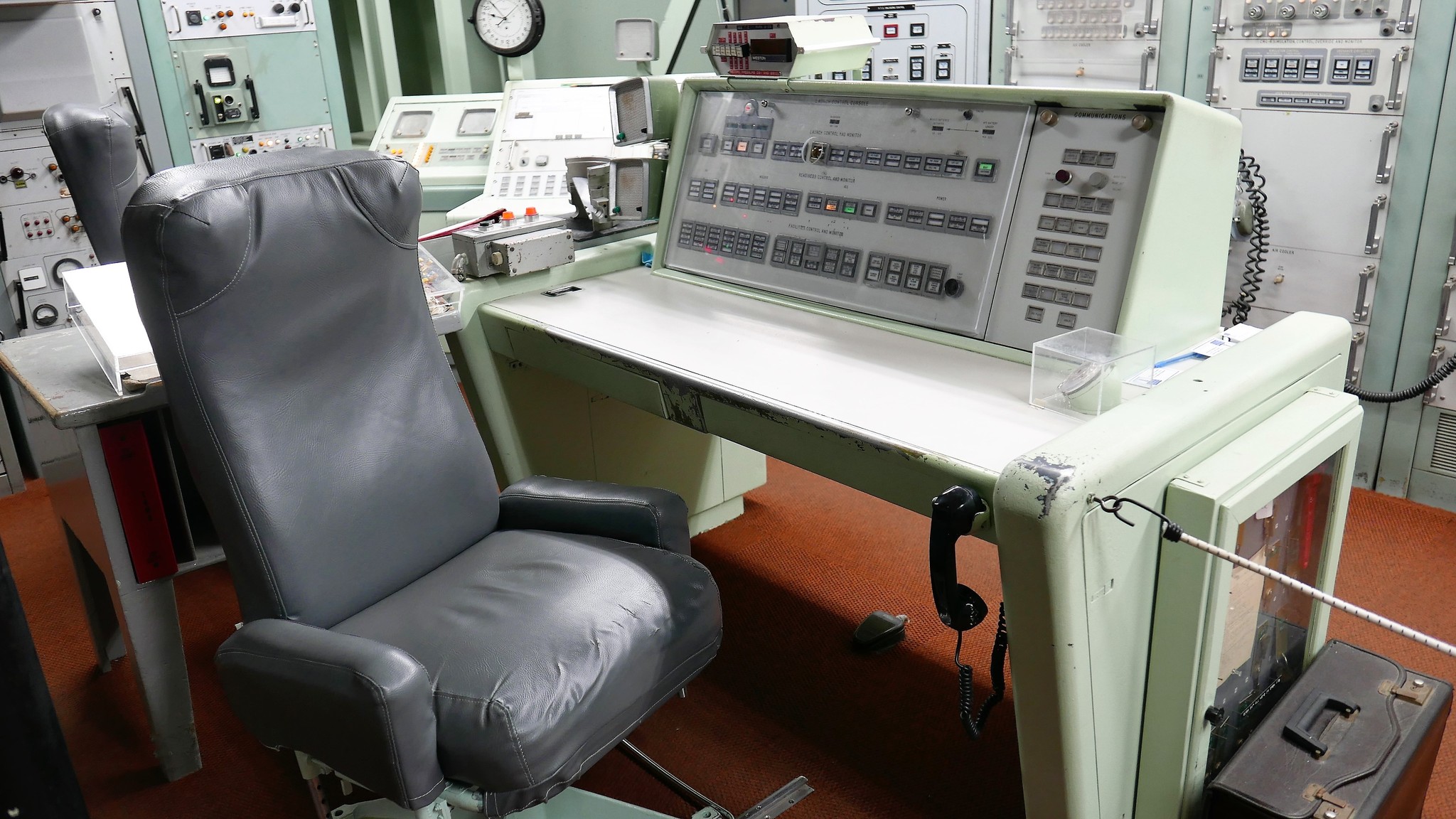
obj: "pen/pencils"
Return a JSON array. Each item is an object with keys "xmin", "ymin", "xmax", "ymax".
[{"xmin": 1155, "ymin": 352, "xmax": 1204, "ymax": 368}]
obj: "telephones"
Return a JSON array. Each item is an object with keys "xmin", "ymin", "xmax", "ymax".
[{"xmin": 928, "ymin": 483, "xmax": 988, "ymax": 630}]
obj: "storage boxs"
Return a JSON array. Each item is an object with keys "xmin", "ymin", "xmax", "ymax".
[
  {"xmin": 1029, "ymin": 326, "xmax": 1156, "ymax": 423},
  {"xmin": 1207, "ymin": 639, "xmax": 1454, "ymax": 819}
]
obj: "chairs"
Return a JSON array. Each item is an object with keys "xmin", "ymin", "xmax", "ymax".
[
  {"xmin": 42, "ymin": 103, "xmax": 139, "ymax": 264},
  {"xmin": 120, "ymin": 147, "xmax": 815, "ymax": 819}
]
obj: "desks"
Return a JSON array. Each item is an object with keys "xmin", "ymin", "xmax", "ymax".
[{"xmin": 0, "ymin": 327, "xmax": 206, "ymax": 780}]
